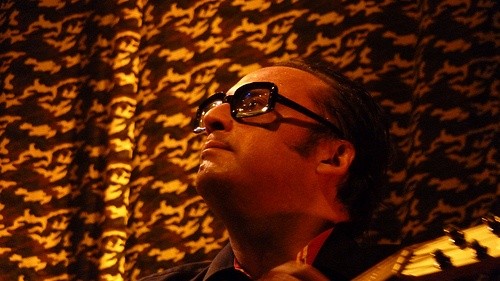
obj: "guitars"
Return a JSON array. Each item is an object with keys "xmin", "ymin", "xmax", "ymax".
[{"xmin": 347, "ymin": 213, "xmax": 500, "ymax": 281}]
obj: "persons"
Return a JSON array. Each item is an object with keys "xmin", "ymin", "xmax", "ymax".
[{"xmin": 133, "ymin": 60, "xmax": 394, "ymax": 281}]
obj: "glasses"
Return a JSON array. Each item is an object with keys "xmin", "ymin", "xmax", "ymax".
[{"xmin": 193, "ymin": 82, "xmax": 343, "ymax": 139}]
